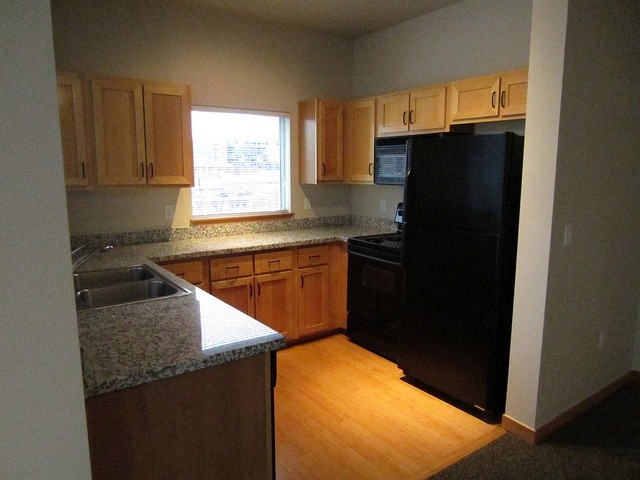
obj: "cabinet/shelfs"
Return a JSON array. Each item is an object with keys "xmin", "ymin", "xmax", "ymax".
[
  {"xmin": 318, "ymin": 101, "xmax": 344, "ymax": 183},
  {"xmin": 452, "ymin": 71, "xmax": 529, "ymax": 121},
  {"xmin": 210, "ymin": 250, "xmax": 295, "ymax": 337},
  {"xmin": 91, "ymin": 78, "xmax": 194, "ymax": 186},
  {"xmin": 55, "ymin": 70, "xmax": 88, "ymax": 186},
  {"xmin": 379, "ymin": 86, "xmax": 446, "ymax": 133},
  {"xmin": 297, "ymin": 246, "xmax": 330, "ymax": 337},
  {"xmin": 346, "ymin": 101, "xmax": 375, "ymax": 184},
  {"xmin": 162, "ymin": 260, "xmax": 204, "ymax": 289}
]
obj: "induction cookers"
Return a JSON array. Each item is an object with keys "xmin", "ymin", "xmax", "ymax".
[{"xmin": 347, "ymin": 202, "xmax": 405, "ymax": 261}]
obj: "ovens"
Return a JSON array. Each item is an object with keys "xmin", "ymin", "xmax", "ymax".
[{"xmin": 346, "ymin": 250, "xmax": 404, "ymax": 362}]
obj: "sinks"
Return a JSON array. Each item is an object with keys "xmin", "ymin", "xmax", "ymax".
[
  {"xmin": 78, "ymin": 280, "xmax": 179, "ymax": 308},
  {"xmin": 76, "ymin": 267, "xmax": 154, "ymax": 290}
]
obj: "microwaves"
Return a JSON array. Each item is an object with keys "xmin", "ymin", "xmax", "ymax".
[{"xmin": 374, "ymin": 145, "xmax": 406, "ymax": 185}]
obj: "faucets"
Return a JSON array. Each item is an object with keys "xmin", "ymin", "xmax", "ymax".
[{"xmin": 72, "ymin": 244, "xmax": 114, "ymax": 274}]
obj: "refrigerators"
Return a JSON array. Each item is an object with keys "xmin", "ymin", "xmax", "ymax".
[{"xmin": 398, "ymin": 131, "xmax": 523, "ymax": 415}]
